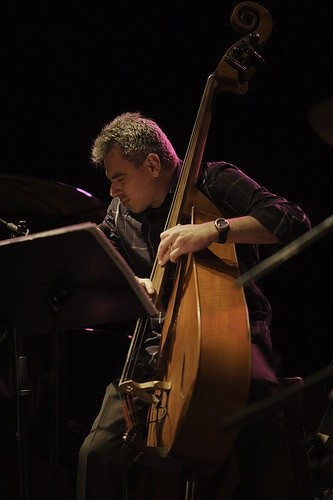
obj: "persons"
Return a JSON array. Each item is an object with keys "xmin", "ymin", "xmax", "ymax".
[{"xmin": 73, "ymin": 110, "xmax": 315, "ymax": 500}]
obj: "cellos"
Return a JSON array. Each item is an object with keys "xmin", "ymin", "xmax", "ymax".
[{"xmin": 119, "ymin": 1, "xmax": 274, "ymax": 500}]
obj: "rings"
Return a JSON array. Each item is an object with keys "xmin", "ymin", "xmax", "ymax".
[{"xmin": 169, "ymin": 245, "xmax": 174, "ymax": 252}]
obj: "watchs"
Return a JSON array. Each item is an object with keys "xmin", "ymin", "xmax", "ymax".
[{"xmin": 214, "ymin": 217, "xmax": 230, "ymax": 244}]
